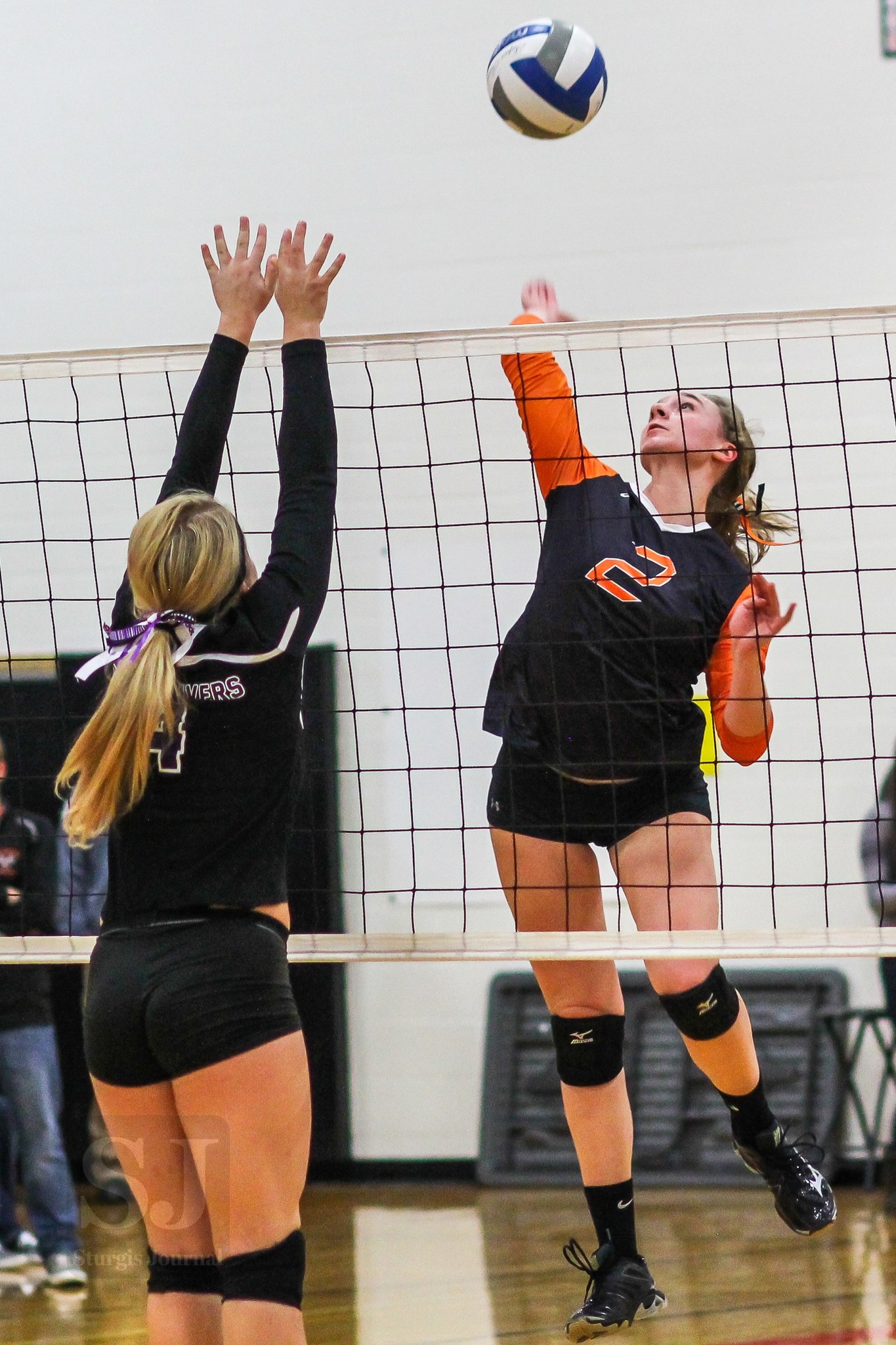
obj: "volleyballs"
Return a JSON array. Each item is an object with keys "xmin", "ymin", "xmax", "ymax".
[{"xmin": 485, "ymin": 18, "xmax": 608, "ymax": 140}]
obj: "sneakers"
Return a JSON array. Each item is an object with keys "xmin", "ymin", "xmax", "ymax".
[
  {"xmin": 731, "ymin": 1119, "xmax": 836, "ymax": 1235},
  {"xmin": 561, "ymin": 1236, "xmax": 667, "ymax": 1344}
]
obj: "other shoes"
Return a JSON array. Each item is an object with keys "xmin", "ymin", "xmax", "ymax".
[
  {"xmin": 44, "ymin": 1250, "xmax": 88, "ymax": 1286},
  {"xmin": 0, "ymin": 1242, "xmax": 27, "ymax": 1269}
]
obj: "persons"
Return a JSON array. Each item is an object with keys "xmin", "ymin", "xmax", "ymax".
[
  {"xmin": 859, "ymin": 743, "xmax": 896, "ymax": 1215},
  {"xmin": 0, "ymin": 740, "xmax": 139, "ymax": 1288},
  {"xmin": 53, "ymin": 215, "xmax": 340, "ymax": 1345},
  {"xmin": 481, "ymin": 281, "xmax": 834, "ymax": 1339}
]
